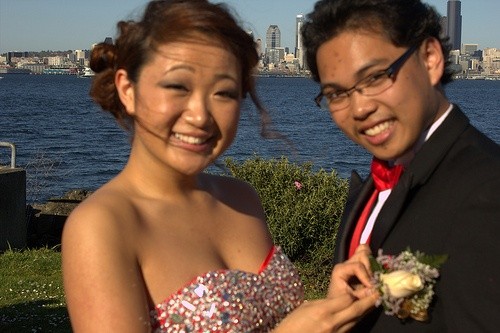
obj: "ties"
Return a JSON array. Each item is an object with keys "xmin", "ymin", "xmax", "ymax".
[{"xmin": 348, "ymin": 154, "xmax": 410, "ymax": 262}]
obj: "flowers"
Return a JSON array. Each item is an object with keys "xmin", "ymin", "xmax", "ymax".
[{"xmin": 349, "ymin": 246, "xmax": 449, "ymax": 323}]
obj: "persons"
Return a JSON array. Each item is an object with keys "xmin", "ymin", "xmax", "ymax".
[
  {"xmin": 299, "ymin": 0, "xmax": 500, "ymax": 333},
  {"xmin": 61, "ymin": 0, "xmax": 381, "ymax": 333}
]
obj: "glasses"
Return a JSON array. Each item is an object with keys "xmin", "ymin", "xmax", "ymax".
[{"xmin": 314, "ymin": 44, "xmax": 419, "ymax": 111}]
